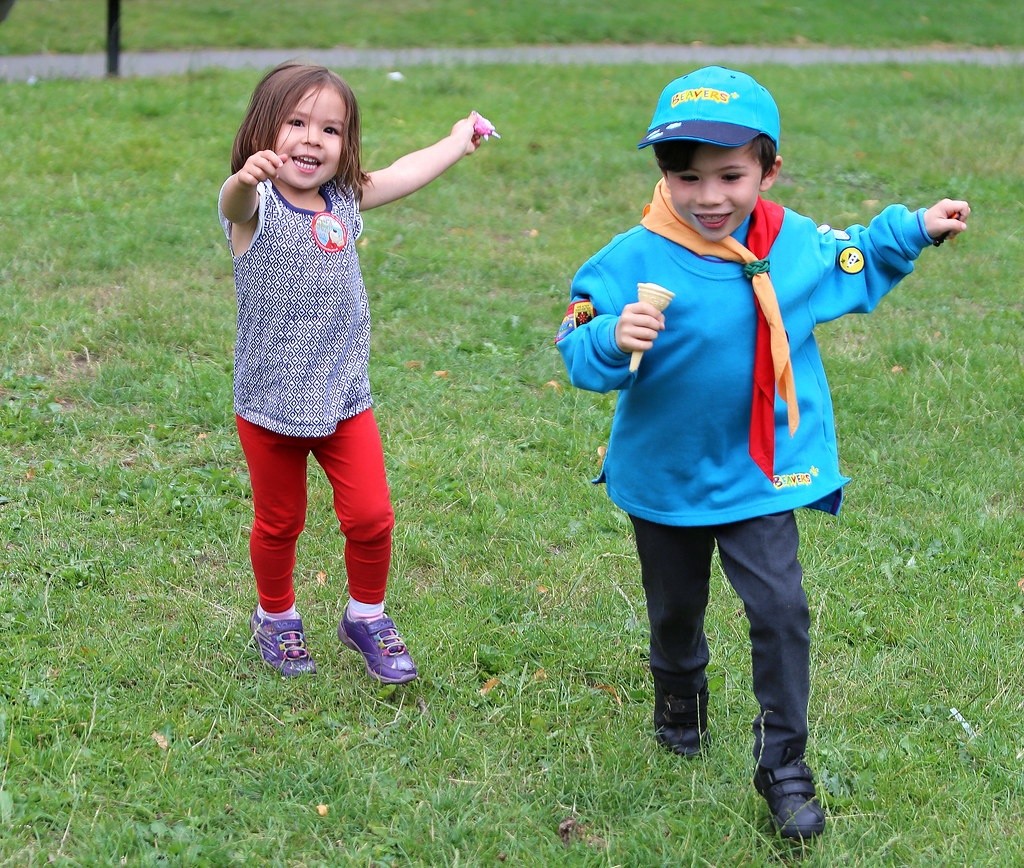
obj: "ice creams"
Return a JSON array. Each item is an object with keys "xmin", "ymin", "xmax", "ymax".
[{"xmin": 629, "ymin": 283, "xmax": 674, "ymax": 372}]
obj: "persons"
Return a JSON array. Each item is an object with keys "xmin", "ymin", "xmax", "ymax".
[
  {"xmin": 553, "ymin": 66, "xmax": 973, "ymax": 837},
  {"xmin": 216, "ymin": 59, "xmax": 482, "ymax": 686}
]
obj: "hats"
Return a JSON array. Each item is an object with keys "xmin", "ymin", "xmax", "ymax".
[{"xmin": 637, "ymin": 64, "xmax": 781, "ymax": 158}]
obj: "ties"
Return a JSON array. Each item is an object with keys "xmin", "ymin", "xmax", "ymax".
[{"xmin": 640, "ymin": 176, "xmax": 800, "ymax": 484}]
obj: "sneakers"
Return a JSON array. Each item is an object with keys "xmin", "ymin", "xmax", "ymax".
[
  {"xmin": 754, "ymin": 754, "xmax": 826, "ymax": 839},
  {"xmin": 250, "ymin": 607, "xmax": 316, "ymax": 679},
  {"xmin": 337, "ymin": 603, "xmax": 418, "ymax": 684},
  {"xmin": 652, "ymin": 672, "xmax": 709, "ymax": 759}
]
